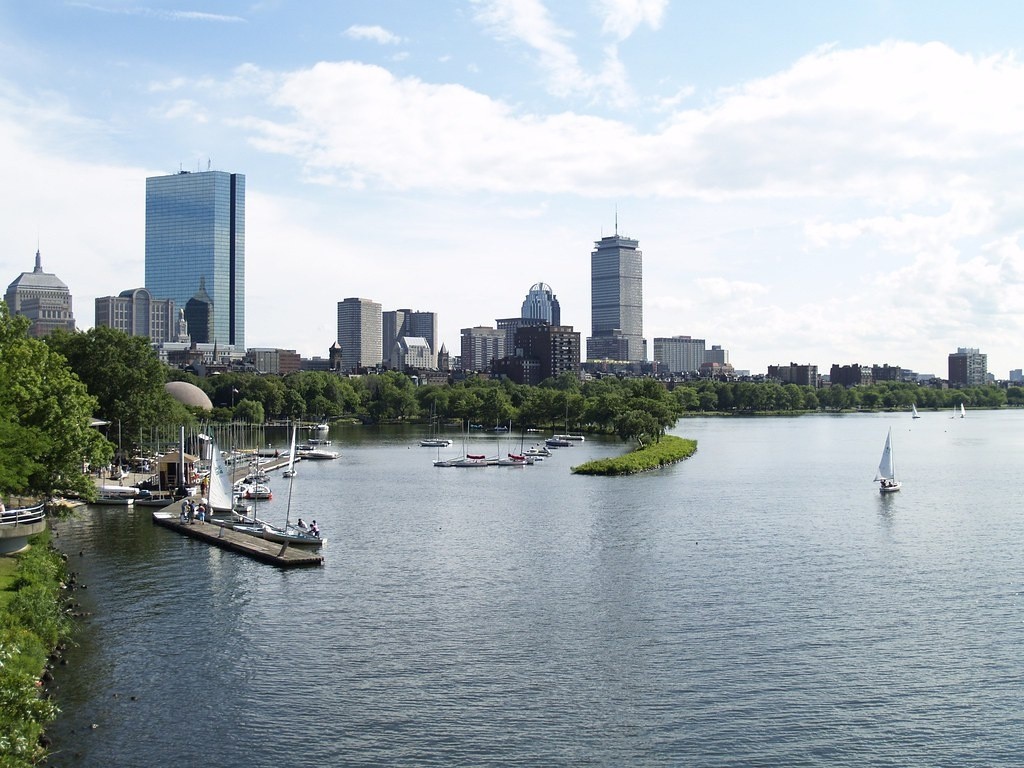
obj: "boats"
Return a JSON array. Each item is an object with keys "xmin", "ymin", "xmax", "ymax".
[
  {"xmin": 233, "ymin": 503, "xmax": 253, "ymax": 512},
  {"xmin": 233, "ymin": 483, "xmax": 250, "ymax": 498},
  {"xmin": 248, "ymin": 481, "xmax": 272, "ymax": 499}
]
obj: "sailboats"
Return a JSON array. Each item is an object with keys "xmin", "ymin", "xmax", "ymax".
[
  {"xmin": 204, "ymin": 437, "xmax": 274, "ymax": 537},
  {"xmin": 911, "ymin": 403, "xmax": 920, "ymax": 419},
  {"xmin": 419, "ymin": 395, "xmax": 586, "ymax": 468},
  {"xmin": 261, "ymin": 440, "xmax": 328, "ymax": 545},
  {"xmin": 245, "ymin": 443, "xmax": 271, "ymax": 484},
  {"xmin": 279, "ymin": 416, "xmax": 339, "ymax": 459},
  {"xmin": 873, "ymin": 425, "xmax": 901, "ymax": 493},
  {"xmin": 81, "ymin": 419, "xmax": 175, "ymax": 505},
  {"xmin": 960, "ymin": 403, "xmax": 966, "ymax": 418}
]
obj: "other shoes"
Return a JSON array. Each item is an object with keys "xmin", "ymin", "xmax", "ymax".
[
  {"xmin": 199, "ymin": 522, "xmax": 201, "ymax": 525},
  {"xmin": 191, "ymin": 522, "xmax": 195, "ymax": 524},
  {"xmin": 202, "ymin": 523, "xmax": 204, "ymax": 525}
]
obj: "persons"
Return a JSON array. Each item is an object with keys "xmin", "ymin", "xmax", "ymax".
[
  {"xmin": 297, "ymin": 519, "xmax": 320, "ymax": 538},
  {"xmin": 275, "ymin": 449, "xmax": 279, "ymax": 462},
  {"xmin": 880, "ymin": 479, "xmax": 892, "ymax": 487},
  {"xmin": 95, "ymin": 462, "xmax": 112, "ymax": 478},
  {"xmin": 181, "ymin": 474, "xmax": 209, "ymax": 526}
]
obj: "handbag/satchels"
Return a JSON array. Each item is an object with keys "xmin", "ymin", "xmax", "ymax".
[{"xmin": 199, "ymin": 506, "xmax": 205, "ymax": 511}]
